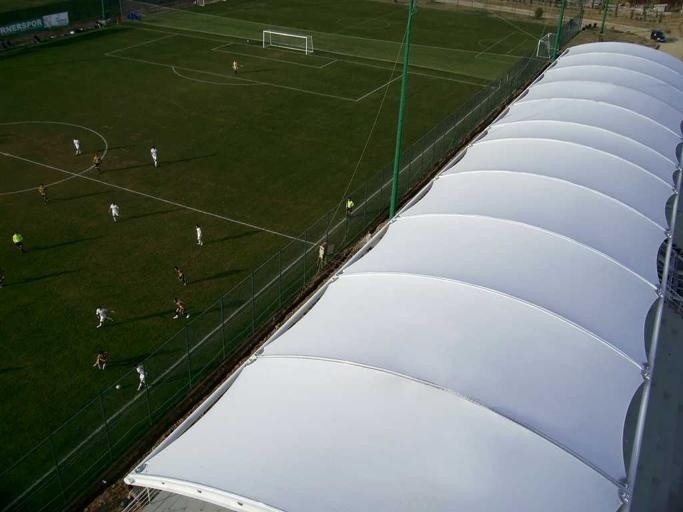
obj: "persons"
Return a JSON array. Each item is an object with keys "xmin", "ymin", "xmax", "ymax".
[
  {"xmin": 93, "ymin": 350, "xmax": 111, "ymax": 370},
  {"xmin": 318, "ymin": 242, "xmax": 327, "ymax": 272},
  {"xmin": 233, "ymin": 60, "xmax": 240, "ymax": 73},
  {"xmin": 194, "ymin": 224, "xmax": 204, "ymax": 247},
  {"xmin": 94, "ymin": 306, "xmax": 115, "ymax": 329},
  {"xmin": 346, "ymin": 195, "xmax": 354, "ymax": 215},
  {"xmin": 172, "ymin": 297, "xmax": 191, "ymax": 319},
  {"xmin": 12, "ymin": 230, "xmax": 26, "ymax": 255},
  {"xmin": 173, "ymin": 265, "xmax": 188, "ymax": 287},
  {"xmin": 38, "ymin": 136, "xmax": 158, "ymax": 223},
  {"xmin": 132, "ymin": 363, "xmax": 150, "ymax": 391},
  {"xmin": 94, "ymin": 14, "xmax": 117, "ymax": 28}
]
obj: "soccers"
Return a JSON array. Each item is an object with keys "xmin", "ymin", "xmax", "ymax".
[{"xmin": 116, "ymin": 385, "xmax": 121, "ymax": 389}]
